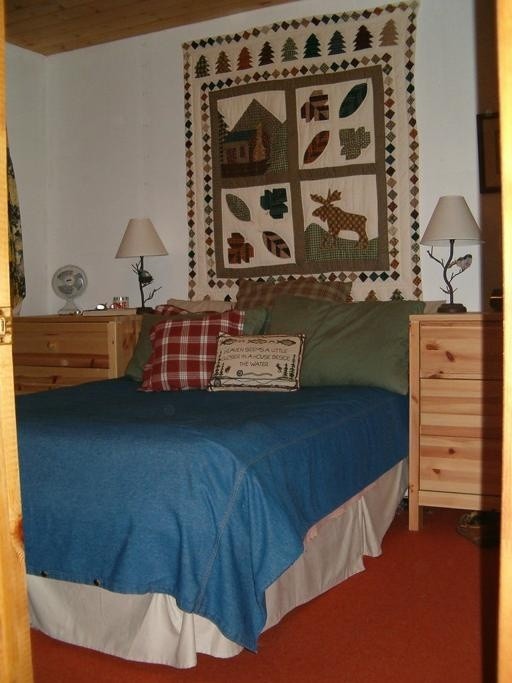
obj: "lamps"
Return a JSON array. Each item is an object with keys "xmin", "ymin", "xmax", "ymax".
[
  {"xmin": 115, "ymin": 218, "xmax": 169, "ymax": 314},
  {"xmin": 420, "ymin": 196, "xmax": 485, "ymax": 313}
]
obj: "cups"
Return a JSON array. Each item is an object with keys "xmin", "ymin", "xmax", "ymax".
[{"xmin": 113, "ymin": 296, "xmax": 129, "ymax": 310}]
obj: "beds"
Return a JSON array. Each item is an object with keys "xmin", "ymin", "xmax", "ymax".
[{"xmin": 15, "ymin": 300, "xmax": 446, "ymax": 668}]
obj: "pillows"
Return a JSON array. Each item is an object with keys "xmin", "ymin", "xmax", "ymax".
[{"xmin": 125, "ymin": 279, "xmax": 425, "ymax": 395}]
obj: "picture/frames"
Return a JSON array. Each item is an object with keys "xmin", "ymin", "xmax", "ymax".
[{"xmin": 477, "ymin": 113, "xmax": 502, "ymax": 195}]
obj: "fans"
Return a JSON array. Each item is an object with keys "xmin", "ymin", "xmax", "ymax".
[{"xmin": 51, "ymin": 264, "xmax": 87, "ymax": 314}]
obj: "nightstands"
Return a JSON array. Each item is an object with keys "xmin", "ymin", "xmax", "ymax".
[
  {"xmin": 408, "ymin": 315, "xmax": 502, "ymax": 531},
  {"xmin": 13, "ymin": 309, "xmax": 142, "ymax": 395}
]
